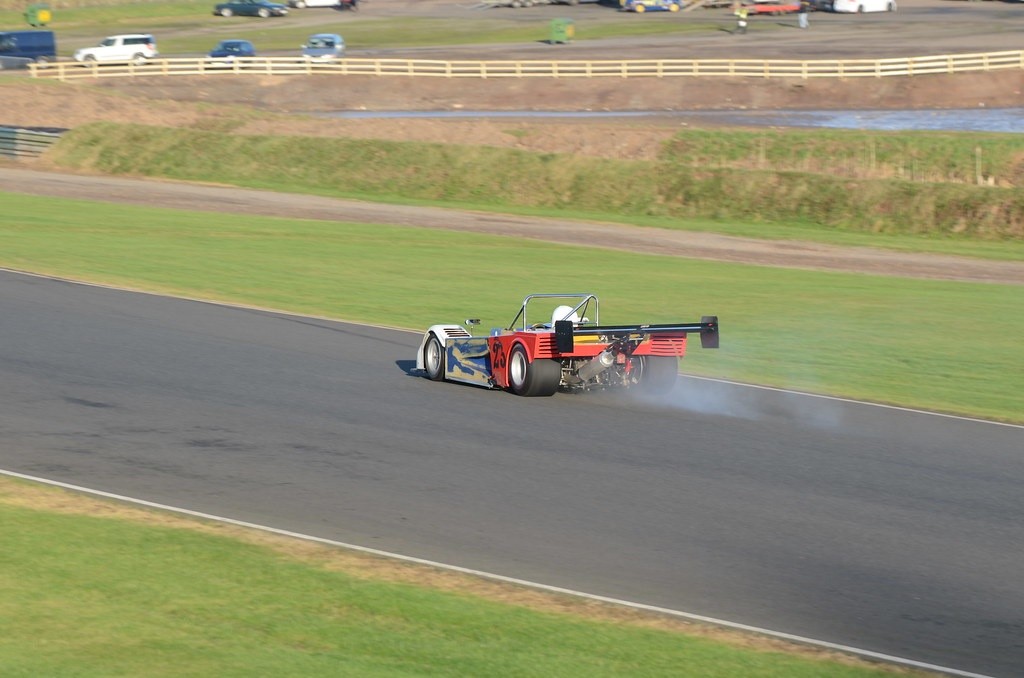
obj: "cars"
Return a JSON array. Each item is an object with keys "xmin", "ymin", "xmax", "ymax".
[
  {"xmin": 620, "ymin": 0, "xmax": 687, "ymax": 14},
  {"xmin": 726, "ymin": 0, "xmax": 898, "ymax": 21},
  {"xmin": 289, "ymin": 0, "xmax": 358, "ymax": 10},
  {"xmin": 203, "ymin": 39, "xmax": 257, "ymax": 71},
  {"xmin": 299, "ymin": 32, "xmax": 348, "ymax": 61},
  {"xmin": 214, "ymin": 0, "xmax": 291, "ymax": 19}
]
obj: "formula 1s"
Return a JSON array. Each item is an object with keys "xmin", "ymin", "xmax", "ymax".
[{"xmin": 415, "ymin": 292, "xmax": 719, "ymax": 397}]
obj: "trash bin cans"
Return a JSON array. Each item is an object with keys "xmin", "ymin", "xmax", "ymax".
[{"xmin": 549, "ymin": 18, "xmax": 576, "ymax": 45}]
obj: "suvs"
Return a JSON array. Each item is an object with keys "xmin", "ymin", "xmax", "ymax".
[{"xmin": 75, "ymin": 32, "xmax": 160, "ymax": 67}]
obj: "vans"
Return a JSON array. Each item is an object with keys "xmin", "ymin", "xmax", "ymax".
[{"xmin": 0, "ymin": 30, "xmax": 57, "ymax": 69}]
